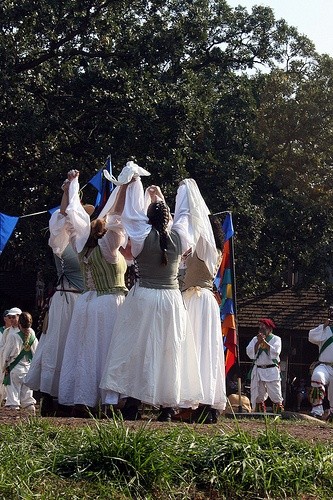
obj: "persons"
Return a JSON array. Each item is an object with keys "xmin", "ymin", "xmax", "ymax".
[
  {"xmin": 307, "ymin": 306, "xmax": 333, "ymax": 423},
  {"xmin": 0, "ymin": 160, "xmax": 309, "ymax": 424}
]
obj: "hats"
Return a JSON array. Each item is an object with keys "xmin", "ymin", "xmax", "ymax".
[
  {"xmin": 4, "ymin": 310, "xmax": 11, "ymax": 317},
  {"xmin": 259, "ymin": 318, "xmax": 275, "ymax": 328},
  {"xmin": 8, "ymin": 307, "xmax": 22, "ymax": 316}
]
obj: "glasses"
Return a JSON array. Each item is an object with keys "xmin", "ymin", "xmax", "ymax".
[{"xmin": 8, "ymin": 315, "xmax": 18, "ymax": 319}]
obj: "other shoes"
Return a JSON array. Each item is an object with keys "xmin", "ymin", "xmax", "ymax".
[
  {"xmin": 40, "ymin": 393, "xmax": 217, "ymax": 423},
  {"xmin": 24, "ymin": 403, "xmax": 36, "ymax": 411},
  {"xmin": 325, "ymin": 412, "xmax": 333, "ymax": 423},
  {"xmin": 11, "ymin": 405, "xmax": 20, "ymax": 411}
]
